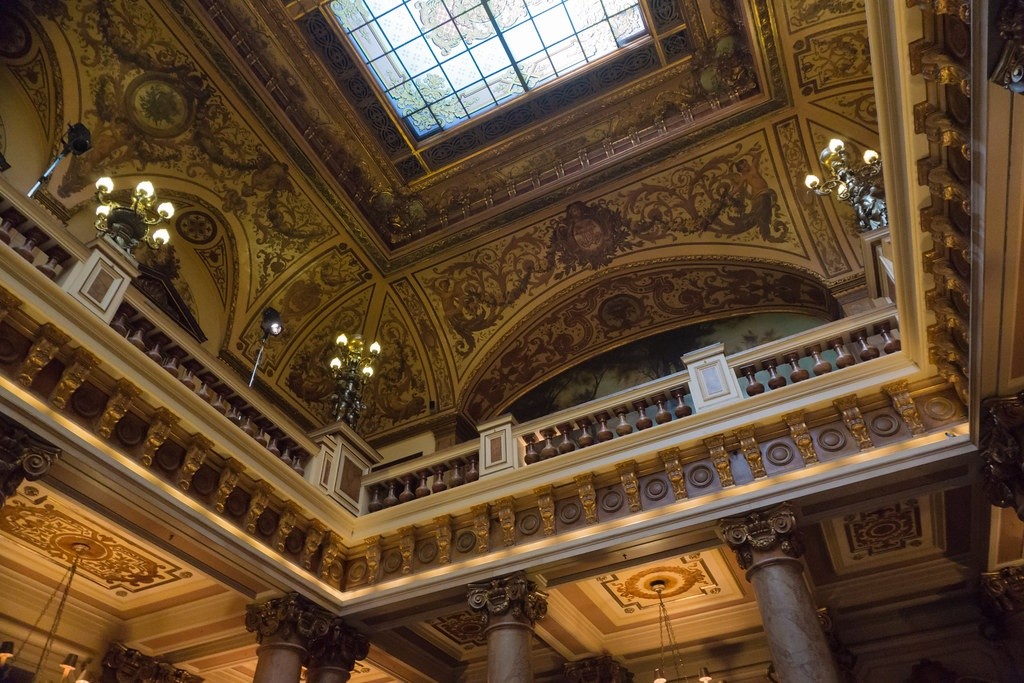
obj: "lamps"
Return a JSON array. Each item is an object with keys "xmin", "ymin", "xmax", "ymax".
[
  {"xmin": 248, "ymin": 306, "xmax": 285, "ymax": 388},
  {"xmin": 26, "ymin": 122, "xmax": 93, "ymax": 198},
  {"xmin": 805, "ymin": 138, "xmax": 889, "ymax": 232},
  {"xmin": 93, "ymin": 176, "xmax": 176, "ymax": 259},
  {"xmin": 0, "ymin": 542, "xmax": 97, "ymax": 683},
  {"xmin": 330, "ymin": 332, "xmax": 381, "ymax": 431},
  {"xmin": 649, "ymin": 579, "xmax": 713, "ymax": 683}
]
